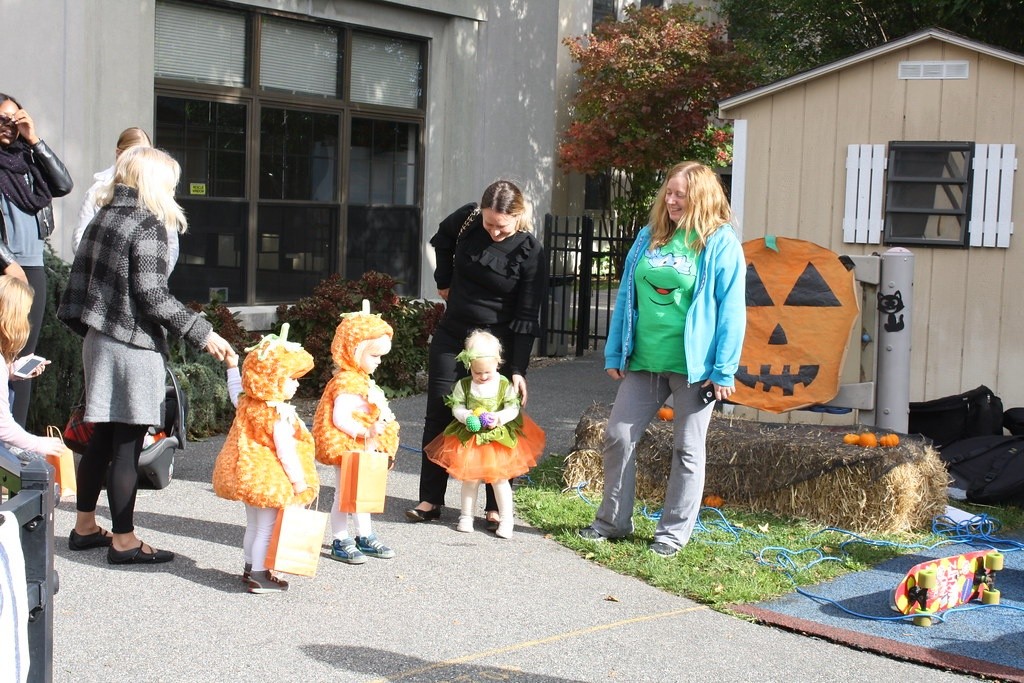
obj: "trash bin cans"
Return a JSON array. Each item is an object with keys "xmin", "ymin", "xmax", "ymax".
[{"xmin": 528, "ymin": 257, "xmax": 575, "ymax": 358}]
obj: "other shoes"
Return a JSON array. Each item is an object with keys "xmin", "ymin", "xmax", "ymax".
[
  {"xmin": 68, "ymin": 527, "xmax": 113, "ymax": 551},
  {"xmin": 485, "ymin": 516, "xmax": 499, "ymax": 531},
  {"xmin": 457, "ymin": 512, "xmax": 475, "ymax": 532},
  {"xmin": 648, "ymin": 541, "xmax": 677, "ymax": 559},
  {"xmin": 406, "ymin": 508, "xmax": 441, "ymax": 521},
  {"xmin": 495, "ymin": 520, "xmax": 514, "ymax": 539},
  {"xmin": 107, "ymin": 540, "xmax": 175, "ymax": 564},
  {"xmin": 575, "ymin": 525, "xmax": 608, "ymax": 543}
]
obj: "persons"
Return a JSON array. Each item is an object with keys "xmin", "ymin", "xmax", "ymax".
[
  {"xmin": 57, "ymin": 146, "xmax": 235, "ymax": 565},
  {"xmin": 422, "ymin": 328, "xmax": 546, "ymax": 539},
  {"xmin": 72, "ymin": 128, "xmax": 152, "ymax": 255},
  {"xmin": 575, "ymin": 160, "xmax": 747, "ymax": 559},
  {"xmin": 312, "ymin": 299, "xmax": 400, "ymax": 564},
  {"xmin": 0, "ymin": 94, "xmax": 74, "ymax": 461},
  {"xmin": 405, "ymin": 180, "xmax": 549, "ymax": 531},
  {"xmin": 212, "ymin": 323, "xmax": 321, "ymax": 594}
]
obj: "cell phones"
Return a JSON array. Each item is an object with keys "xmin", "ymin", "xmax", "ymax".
[
  {"xmin": 14, "ymin": 356, "xmax": 46, "ymax": 378},
  {"xmin": 699, "ymin": 384, "xmax": 716, "ymax": 405}
]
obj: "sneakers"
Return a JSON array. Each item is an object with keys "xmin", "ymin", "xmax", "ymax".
[
  {"xmin": 355, "ymin": 535, "xmax": 395, "ymax": 559},
  {"xmin": 248, "ymin": 570, "xmax": 289, "ymax": 594},
  {"xmin": 243, "ymin": 563, "xmax": 252, "ymax": 582},
  {"xmin": 330, "ymin": 539, "xmax": 368, "ymax": 564}
]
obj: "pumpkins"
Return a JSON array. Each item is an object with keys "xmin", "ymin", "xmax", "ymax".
[
  {"xmin": 704, "ymin": 493, "xmax": 722, "ymax": 507},
  {"xmin": 844, "ymin": 434, "xmax": 860, "ymax": 445},
  {"xmin": 858, "ymin": 429, "xmax": 878, "ymax": 448},
  {"xmin": 657, "ymin": 405, "xmax": 674, "ymax": 421},
  {"xmin": 879, "ymin": 432, "xmax": 899, "ymax": 448}
]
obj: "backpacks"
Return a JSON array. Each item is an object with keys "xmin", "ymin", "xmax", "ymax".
[{"xmin": 941, "ymin": 433, "xmax": 1023, "ymax": 504}]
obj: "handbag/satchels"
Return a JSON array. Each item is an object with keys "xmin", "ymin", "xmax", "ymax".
[
  {"xmin": 264, "ymin": 485, "xmax": 327, "ymax": 577},
  {"xmin": 908, "ymin": 386, "xmax": 1004, "ymax": 449},
  {"xmin": 63, "ymin": 390, "xmax": 97, "ymax": 453},
  {"xmin": 46, "ymin": 425, "xmax": 77, "ymax": 497},
  {"xmin": 338, "ymin": 431, "xmax": 389, "ymax": 513}
]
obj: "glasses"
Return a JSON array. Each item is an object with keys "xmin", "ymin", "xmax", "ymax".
[{"xmin": 0, "ymin": 116, "xmax": 20, "ymax": 130}]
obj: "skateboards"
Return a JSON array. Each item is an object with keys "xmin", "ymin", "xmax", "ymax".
[{"xmin": 894, "ymin": 548, "xmax": 1003, "ymax": 626}]
